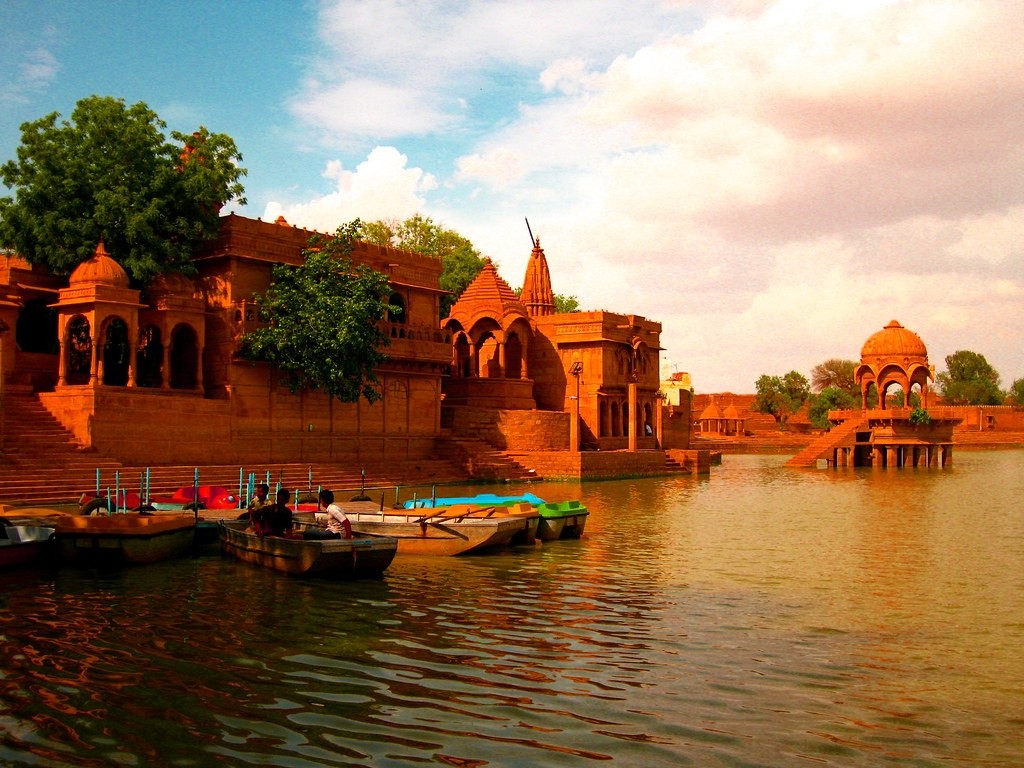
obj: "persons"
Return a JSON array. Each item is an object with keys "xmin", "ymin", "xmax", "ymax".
[
  {"xmin": 646, "ymin": 425, "xmax": 652, "ymax": 435},
  {"xmin": 303, "ymin": 489, "xmax": 352, "ymax": 540},
  {"xmin": 237, "ymin": 484, "xmax": 293, "ymax": 537}
]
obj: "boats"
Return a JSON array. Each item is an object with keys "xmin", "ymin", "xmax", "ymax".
[
  {"xmin": 215, "ymin": 518, "xmax": 398, "ymax": 582},
  {"xmin": 404, "ymin": 493, "xmax": 590, "ymax": 545},
  {"xmin": 1, "ymin": 466, "xmax": 327, "ymax": 569},
  {"xmin": 290, "ymin": 509, "xmax": 528, "ymax": 557}
]
